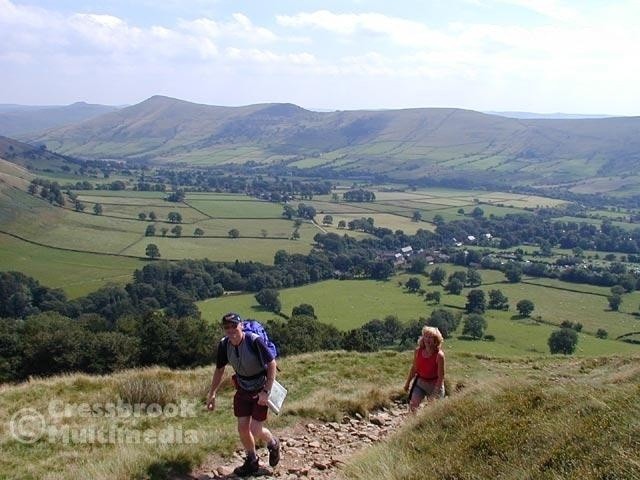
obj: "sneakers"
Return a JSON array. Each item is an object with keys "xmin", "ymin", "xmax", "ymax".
[
  {"xmin": 267, "ymin": 436, "xmax": 280, "ymax": 466},
  {"xmin": 234, "ymin": 457, "xmax": 259, "ymax": 476}
]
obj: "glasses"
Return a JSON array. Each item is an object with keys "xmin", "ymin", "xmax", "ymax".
[{"xmin": 224, "ymin": 324, "xmax": 237, "ymax": 329}]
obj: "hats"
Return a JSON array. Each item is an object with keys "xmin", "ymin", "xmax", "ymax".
[{"xmin": 222, "ymin": 314, "xmax": 241, "ymax": 326}]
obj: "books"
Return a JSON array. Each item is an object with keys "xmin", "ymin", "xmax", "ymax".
[{"xmin": 264, "ymin": 378, "xmax": 288, "ymax": 414}]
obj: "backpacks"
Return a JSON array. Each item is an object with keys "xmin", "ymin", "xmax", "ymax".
[{"xmin": 223, "ymin": 320, "xmax": 276, "ymax": 370}]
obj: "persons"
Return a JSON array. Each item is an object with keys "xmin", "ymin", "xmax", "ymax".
[
  {"xmin": 403, "ymin": 325, "xmax": 446, "ymax": 417},
  {"xmin": 205, "ymin": 314, "xmax": 282, "ymax": 475}
]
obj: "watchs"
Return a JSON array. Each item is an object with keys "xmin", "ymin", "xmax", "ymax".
[{"xmin": 263, "ymin": 388, "xmax": 271, "ymax": 395}]
